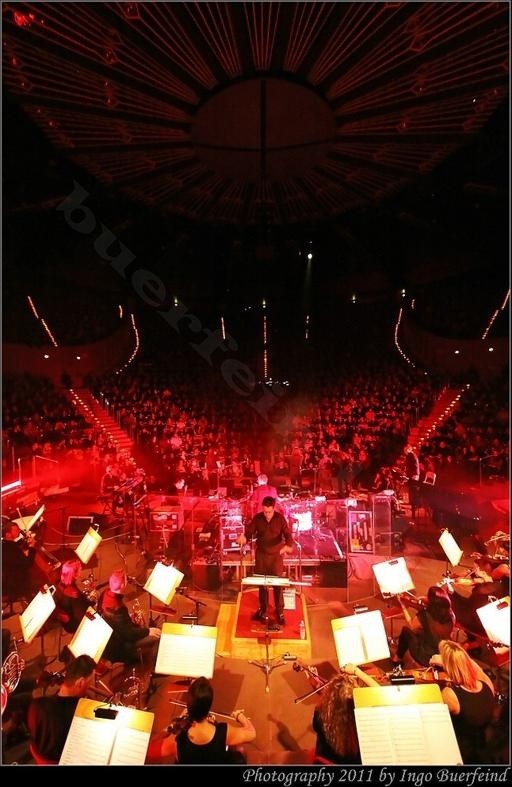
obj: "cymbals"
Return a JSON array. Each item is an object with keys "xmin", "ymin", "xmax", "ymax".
[
  {"xmin": 278, "ymin": 484, "xmax": 301, "ymax": 491},
  {"xmin": 241, "ymin": 479, "xmax": 260, "ymax": 486}
]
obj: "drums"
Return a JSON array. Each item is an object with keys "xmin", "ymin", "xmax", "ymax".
[
  {"xmin": 220, "ymin": 514, "xmax": 244, "ymax": 528},
  {"xmin": 291, "ymin": 507, "xmax": 312, "ymax": 531}
]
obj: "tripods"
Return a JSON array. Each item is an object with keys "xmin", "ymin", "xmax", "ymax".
[
  {"xmin": 247, "ymin": 589, "xmax": 287, "ymax": 693},
  {"xmin": 26, "ymin": 625, "xmax": 57, "ymax": 676}
]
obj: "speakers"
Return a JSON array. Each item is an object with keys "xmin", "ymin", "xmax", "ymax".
[
  {"xmin": 67, "ymin": 516, "xmax": 94, "ymax": 535},
  {"xmin": 375, "ymin": 531, "xmax": 405, "ymax": 555},
  {"xmin": 150, "ymin": 506, "xmax": 184, "ymax": 532}
]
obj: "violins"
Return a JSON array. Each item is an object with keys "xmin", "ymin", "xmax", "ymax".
[
  {"xmin": 3, "ymin": 509, "xmax": 238, "ymax": 767},
  {"xmin": 285, "ymin": 525, "xmax": 508, "ymax": 764}
]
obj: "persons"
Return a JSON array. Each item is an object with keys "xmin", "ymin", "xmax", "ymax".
[{"xmin": 1, "ymin": 354, "xmax": 509, "ymax": 765}]
